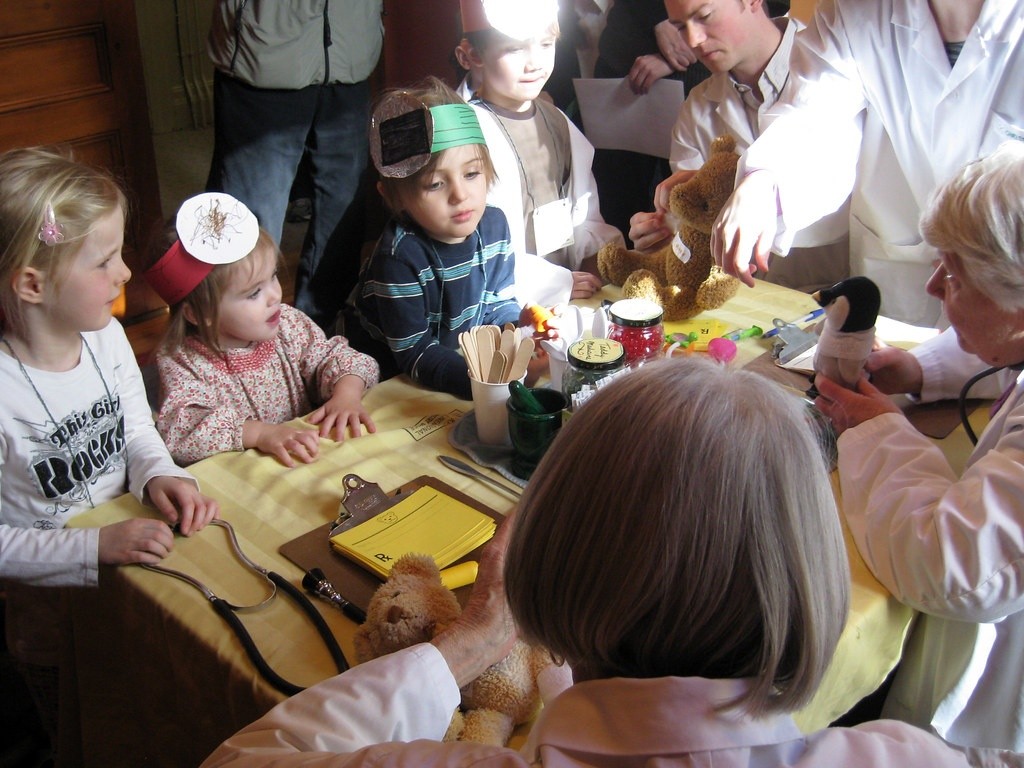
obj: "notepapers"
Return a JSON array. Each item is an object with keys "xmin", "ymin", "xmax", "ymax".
[{"xmin": 329, "ymin": 485, "xmax": 495, "ymax": 577}]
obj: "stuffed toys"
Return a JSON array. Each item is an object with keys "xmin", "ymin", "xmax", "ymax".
[
  {"xmin": 811, "ymin": 276, "xmax": 881, "ymax": 387},
  {"xmin": 597, "ymin": 135, "xmax": 740, "ymax": 323},
  {"xmin": 353, "ymin": 554, "xmax": 564, "ymax": 748}
]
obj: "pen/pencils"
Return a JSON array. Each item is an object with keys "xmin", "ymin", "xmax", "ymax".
[{"xmin": 760, "ymin": 308, "xmax": 824, "ymax": 340}]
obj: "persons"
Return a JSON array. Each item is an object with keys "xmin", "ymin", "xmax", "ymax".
[
  {"xmin": 811, "ymin": 142, "xmax": 1024, "ymax": 768},
  {"xmin": 0, "ymin": 149, "xmax": 215, "ymax": 747},
  {"xmin": 188, "ymin": 0, "xmax": 1024, "ymax": 388},
  {"xmin": 201, "ymin": 359, "xmax": 972, "ymax": 768},
  {"xmin": 147, "ymin": 190, "xmax": 380, "ymax": 467}
]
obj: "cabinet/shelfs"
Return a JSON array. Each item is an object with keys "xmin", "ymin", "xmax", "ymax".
[{"xmin": 0, "ymin": 0, "xmax": 168, "ymax": 318}]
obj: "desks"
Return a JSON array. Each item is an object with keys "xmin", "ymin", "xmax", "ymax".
[{"xmin": 62, "ymin": 275, "xmax": 1000, "ymax": 746}]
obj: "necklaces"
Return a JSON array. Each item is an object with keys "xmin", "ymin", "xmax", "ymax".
[{"xmin": 0, "ymin": 331, "xmax": 129, "ymax": 510}]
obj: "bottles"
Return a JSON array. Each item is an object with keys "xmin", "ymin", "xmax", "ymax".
[
  {"xmin": 559, "ymin": 337, "xmax": 627, "ymax": 414},
  {"xmin": 605, "ymin": 298, "xmax": 665, "ymax": 368}
]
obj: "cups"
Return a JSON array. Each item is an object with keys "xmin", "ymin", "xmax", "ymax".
[{"xmin": 467, "ymin": 366, "xmax": 528, "ymax": 446}]
